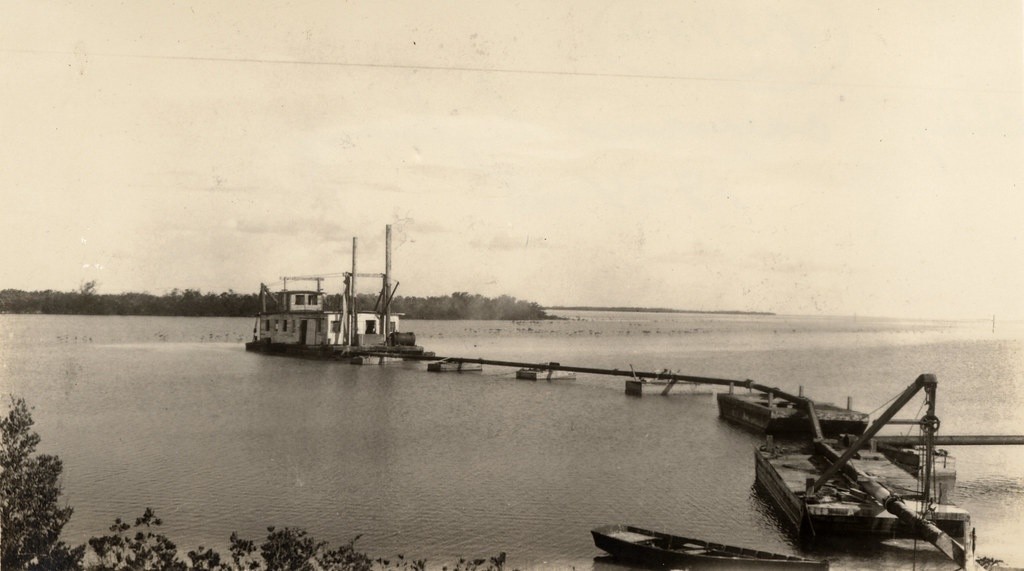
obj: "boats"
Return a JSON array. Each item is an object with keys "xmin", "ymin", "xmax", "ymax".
[
  {"xmin": 756, "ymin": 373, "xmax": 971, "ymax": 554},
  {"xmin": 245, "ymin": 224, "xmax": 436, "ymax": 363},
  {"xmin": 590, "ymin": 523, "xmax": 829, "ymax": 571},
  {"xmin": 718, "ymin": 385, "xmax": 871, "ymax": 440}
]
